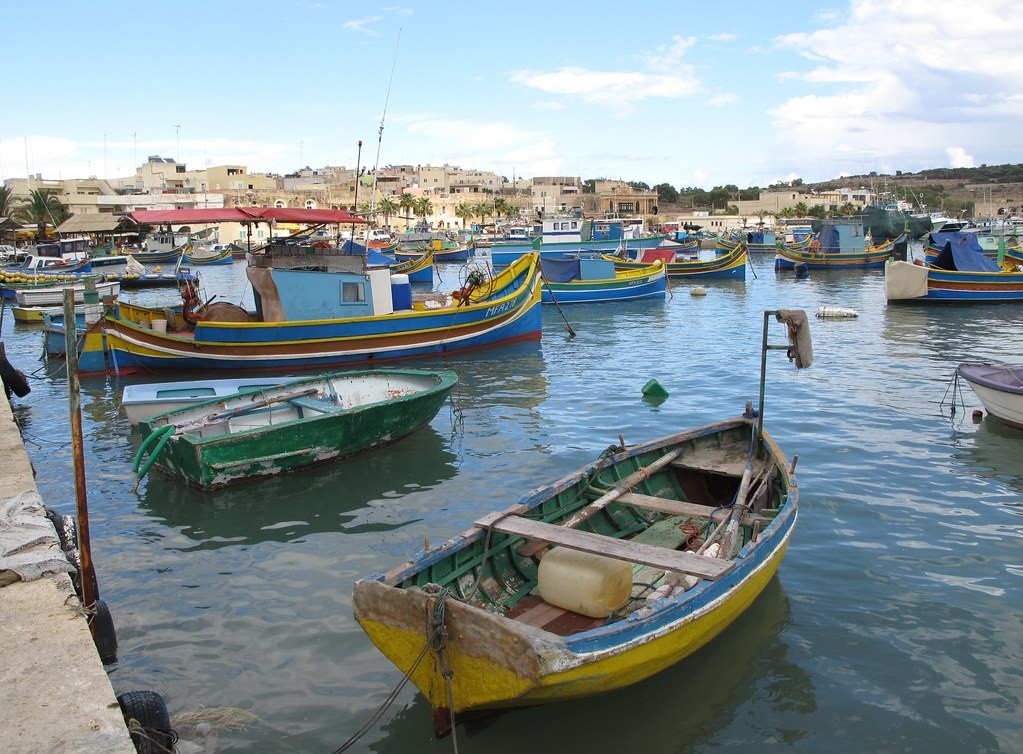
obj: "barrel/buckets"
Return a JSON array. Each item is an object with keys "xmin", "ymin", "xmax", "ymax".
[
  {"xmin": 690, "ymin": 287, "xmax": 707, "ymax": 295},
  {"xmin": 538, "ymin": 546, "xmax": 633, "ymax": 618},
  {"xmin": 151, "ymin": 319, "xmax": 167, "ymax": 334},
  {"xmin": 641, "ymin": 379, "xmax": 669, "ymax": 397},
  {"xmin": 794, "ymin": 263, "xmax": 809, "ymax": 279},
  {"xmin": 391, "ymin": 273, "xmax": 412, "ymax": 311}
]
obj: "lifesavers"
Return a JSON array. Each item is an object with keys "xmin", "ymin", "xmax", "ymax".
[
  {"xmin": 117, "ymin": 691, "xmax": 174, "ymax": 754},
  {"xmin": 71, "ymin": 551, "xmax": 99, "ymax": 600},
  {"xmin": 91, "ymin": 600, "xmax": 116, "ymax": 658},
  {"xmin": 0, "ymin": 354, "xmax": 31, "ymax": 398},
  {"xmin": 53, "ymin": 514, "xmax": 78, "ymax": 552}
]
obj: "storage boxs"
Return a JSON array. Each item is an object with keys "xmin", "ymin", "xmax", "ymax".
[{"xmin": 266, "ymin": 244, "xmax": 346, "ymax": 255}]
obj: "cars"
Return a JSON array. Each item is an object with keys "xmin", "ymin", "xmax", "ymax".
[{"xmin": 0, "ymin": 245, "xmax": 29, "ymax": 262}]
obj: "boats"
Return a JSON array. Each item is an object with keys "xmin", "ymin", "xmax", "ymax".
[
  {"xmin": 0, "ymin": 235, "xmax": 188, "ymax": 300},
  {"xmin": 487, "ymin": 216, "xmax": 749, "ymax": 303},
  {"xmin": 187, "ymin": 248, "xmax": 233, "ymax": 265},
  {"xmin": 886, "ymin": 257, "xmax": 1023, "ymax": 302},
  {"xmin": 718, "ymin": 202, "xmax": 1023, "ymax": 267},
  {"xmin": 957, "ymin": 362, "xmax": 1023, "ymax": 430},
  {"xmin": 14, "ymin": 281, "xmax": 120, "ymax": 305},
  {"xmin": 122, "ymin": 376, "xmax": 318, "ymax": 425},
  {"xmin": 353, "ymin": 309, "xmax": 805, "ymax": 718},
  {"xmin": 44, "ymin": 304, "xmax": 195, "ymax": 353},
  {"xmin": 78, "ymin": 206, "xmax": 544, "ymax": 374},
  {"xmin": 10, "ymin": 301, "xmax": 103, "ymax": 321},
  {"xmin": 345, "ymin": 222, "xmax": 478, "ymax": 282},
  {"xmin": 139, "ymin": 366, "xmax": 460, "ymax": 488}
]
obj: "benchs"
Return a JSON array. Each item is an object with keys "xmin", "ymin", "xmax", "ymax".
[
  {"xmin": 279, "ymin": 394, "xmax": 344, "ymax": 412},
  {"xmin": 473, "ymin": 511, "xmax": 736, "ymax": 586},
  {"xmin": 593, "ymin": 484, "xmax": 763, "ymax": 532}
]
